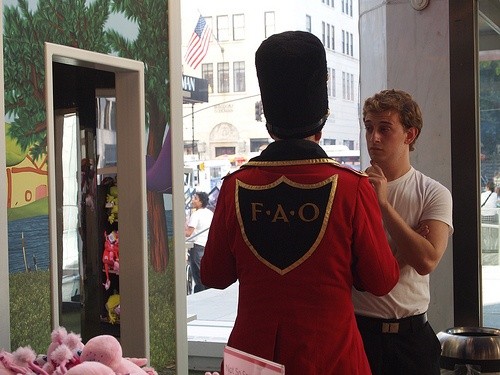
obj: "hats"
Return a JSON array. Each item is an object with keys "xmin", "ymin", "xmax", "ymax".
[{"xmin": 255, "ymin": 31, "xmax": 333, "ymax": 138}]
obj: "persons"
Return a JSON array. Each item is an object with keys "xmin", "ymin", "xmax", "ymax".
[
  {"xmin": 200, "ymin": 31, "xmax": 400, "ymax": 375},
  {"xmin": 494, "ymin": 186, "xmax": 500, "ymax": 208},
  {"xmin": 185, "ymin": 192, "xmax": 214, "ymax": 293},
  {"xmin": 481, "ymin": 181, "xmax": 499, "ymax": 224},
  {"xmin": 352, "ymin": 88, "xmax": 454, "ymax": 375}
]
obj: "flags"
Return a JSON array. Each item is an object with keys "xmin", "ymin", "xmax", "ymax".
[{"xmin": 184, "ymin": 15, "xmax": 213, "ymax": 70}]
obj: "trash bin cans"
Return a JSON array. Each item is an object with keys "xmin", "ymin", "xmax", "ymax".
[{"xmin": 437, "ymin": 325, "xmax": 500, "ymax": 373}]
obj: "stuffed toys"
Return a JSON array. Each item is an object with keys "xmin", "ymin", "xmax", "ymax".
[
  {"xmin": 0, "ymin": 326, "xmax": 220, "ymax": 375},
  {"xmin": 105, "ymin": 294, "xmax": 120, "ymax": 325},
  {"xmin": 106, "ymin": 183, "xmax": 119, "ymax": 224},
  {"xmin": 103, "ymin": 229, "xmax": 119, "ymax": 290}
]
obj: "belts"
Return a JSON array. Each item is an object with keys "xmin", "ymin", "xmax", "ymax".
[{"xmin": 356, "ymin": 313, "xmax": 429, "ymax": 334}]
